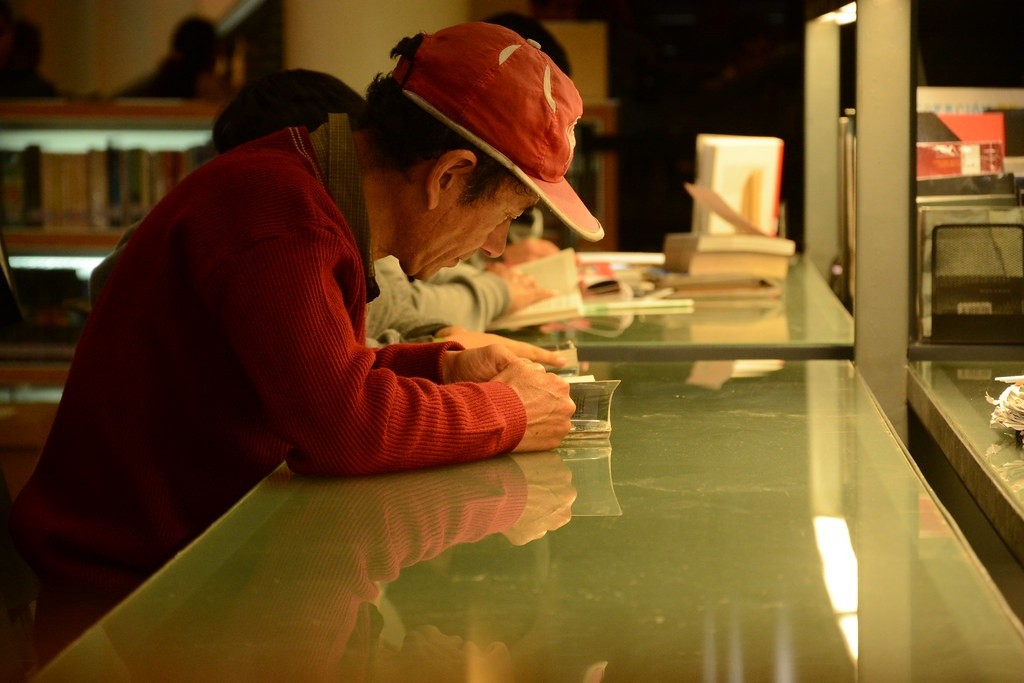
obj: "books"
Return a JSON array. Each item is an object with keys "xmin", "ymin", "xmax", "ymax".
[
  {"xmin": 562, "ymin": 375, "xmax": 622, "ymax": 432},
  {"xmin": 644, "ymin": 233, "xmax": 796, "ymax": 308},
  {"xmin": 532, "ymin": 341, "xmax": 580, "ymax": 376},
  {"xmin": 484, "ymin": 247, "xmax": 584, "ymax": 332},
  {"xmin": 1, "ymin": 146, "xmax": 211, "ymax": 232}
]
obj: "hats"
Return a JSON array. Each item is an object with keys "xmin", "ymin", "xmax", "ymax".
[{"xmin": 392, "ymin": 22, "xmax": 605, "ymax": 242}]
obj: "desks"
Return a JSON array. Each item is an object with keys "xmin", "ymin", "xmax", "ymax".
[
  {"xmin": 490, "ymin": 250, "xmax": 856, "ymax": 360},
  {"xmin": 906, "ymin": 360, "xmax": 1022, "ymax": 623},
  {"xmin": 28, "ymin": 361, "xmax": 1023, "ymax": 683}
]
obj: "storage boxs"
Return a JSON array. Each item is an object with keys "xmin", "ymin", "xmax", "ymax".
[{"xmin": 916, "ymin": 110, "xmax": 1007, "ymax": 181}]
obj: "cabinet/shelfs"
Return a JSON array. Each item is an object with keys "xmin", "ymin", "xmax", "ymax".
[{"xmin": 0, "ymin": 0, "xmax": 286, "ymax": 447}]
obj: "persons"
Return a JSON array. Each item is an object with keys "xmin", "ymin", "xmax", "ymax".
[
  {"xmin": 1, "ymin": 11, "xmax": 66, "ymax": 100},
  {"xmin": 99, "ymin": 449, "xmax": 608, "ymax": 683},
  {"xmin": 143, "ymin": 16, "xmax": 229, "ymax": 98},
  {"xmin": 1, "ymin": 13, "xmax": 583, "ymax": 683}
]
